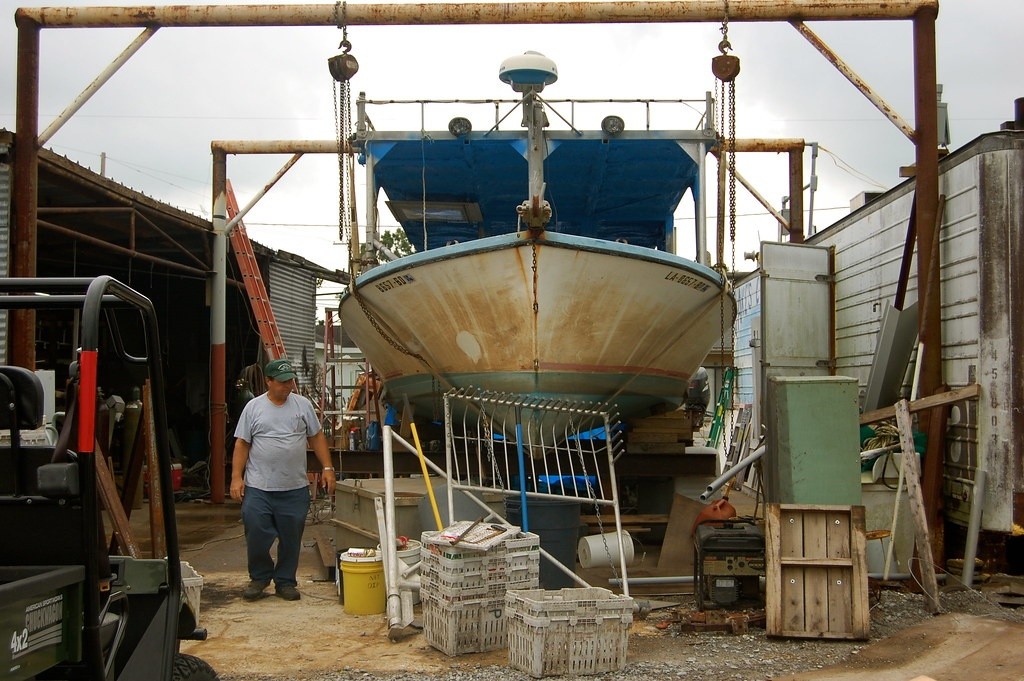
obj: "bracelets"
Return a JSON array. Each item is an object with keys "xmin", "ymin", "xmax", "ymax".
[{"xmin": 324, "ymin": 467, "xmax": 334, "ymax": 471}]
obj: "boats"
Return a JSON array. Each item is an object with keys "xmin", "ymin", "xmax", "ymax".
[{"xmin": 336, "ymin": 48, "xmax": 739, "ymax": 465}]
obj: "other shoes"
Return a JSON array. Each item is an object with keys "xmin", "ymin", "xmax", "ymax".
[
  {"xmin": 243, "ymin": 578, "xmax": 272, "ymax": 600},
  {"xmin": 275, "ymin": 583, "xmax": 300, "ymax": 600}
]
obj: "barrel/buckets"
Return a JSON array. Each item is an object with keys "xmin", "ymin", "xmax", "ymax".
[
  {"xmin": 867, "ymin": 530, "xmax": 898, "ymax": 574},
  {"xmin": 376, "ymin": 539, "xmax": 422, "ymax": 604},
  {"xmin": 578, "ymin": 530, "xmax": 646, "ymax": 569},
  {"xmin": 340, "ymin": 549, "xmax": 386, "ymax": 615}
]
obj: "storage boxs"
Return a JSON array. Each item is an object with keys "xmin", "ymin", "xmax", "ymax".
[
  {"xmin": 179, "ymin": 561, "xmax": 204, "ymax": 627},
  {"xmin": 419, "ymin": 530, "xmax": 635, "ymax": 679}
]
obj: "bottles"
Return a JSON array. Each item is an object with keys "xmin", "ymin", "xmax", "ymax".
[{"xmin": 350, "ymin": 431, "xmax": 355, "ymax": 451}]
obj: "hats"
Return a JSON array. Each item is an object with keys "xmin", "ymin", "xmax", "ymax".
[{"xmin": 265, "ymin": 359, "xmax": 298, "ymax": 382}]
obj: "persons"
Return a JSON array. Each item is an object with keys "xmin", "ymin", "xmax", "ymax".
[{"xmin": 229, "ymin": 359, "xmax": 336, "ymax": 601}]
